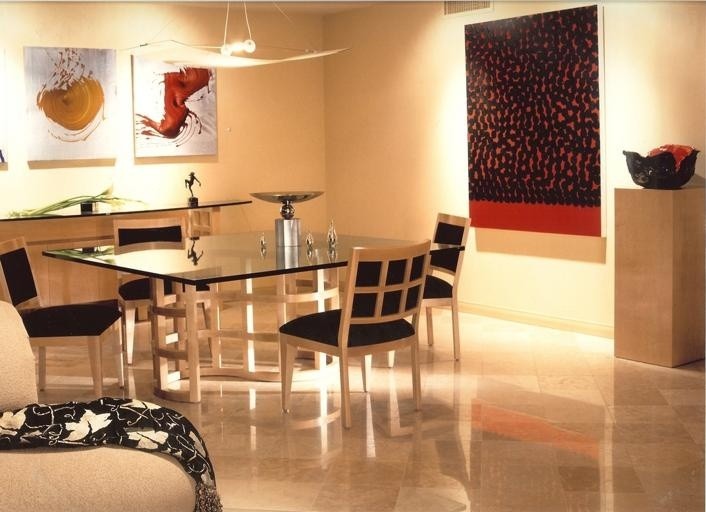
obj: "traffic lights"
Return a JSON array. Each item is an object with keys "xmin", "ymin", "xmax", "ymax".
[{"xmin": 0, "ymin": 300, "xmax": 38, "ymax": 413}]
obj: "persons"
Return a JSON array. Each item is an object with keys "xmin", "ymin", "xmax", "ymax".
[
  {"xmin": 187, "ymin": 240, "xmax": 205, "ymax": 266},
  {"xmin": 185, "ymin": 171, "xmax": 201, "ymax": 197}
]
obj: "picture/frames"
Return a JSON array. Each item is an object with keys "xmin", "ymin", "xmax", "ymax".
[
  {"xmin": 41, "ymin": 225, "xmax": 464, "ymax": 402},
  {"xmin": 0, "ymin": 198, "xmax": 253, "ymax": 308}
]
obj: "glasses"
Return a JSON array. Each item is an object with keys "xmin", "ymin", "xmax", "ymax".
[{"xmin": 129, "ymin": 1, "xmax": 350, "ymax": 69}]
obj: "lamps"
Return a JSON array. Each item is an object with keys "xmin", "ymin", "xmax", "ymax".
[
  {"xmin": 278, "ymin": 241, "xmax": 432, "ymax": 429},
  {"xmin": 421, "ymin": 213, "xmax": 472, "ymax": 360},
  {"xmin": 111, "ymin": 217, "xmax": 220, "ymax": 364},
  {"xmin": 0, "ymin": 235, "xmax": 124, "ymax": 398},
  {"xmin": 0, "ymin": 300, "xmax": 199, "ymax": 512}
]
